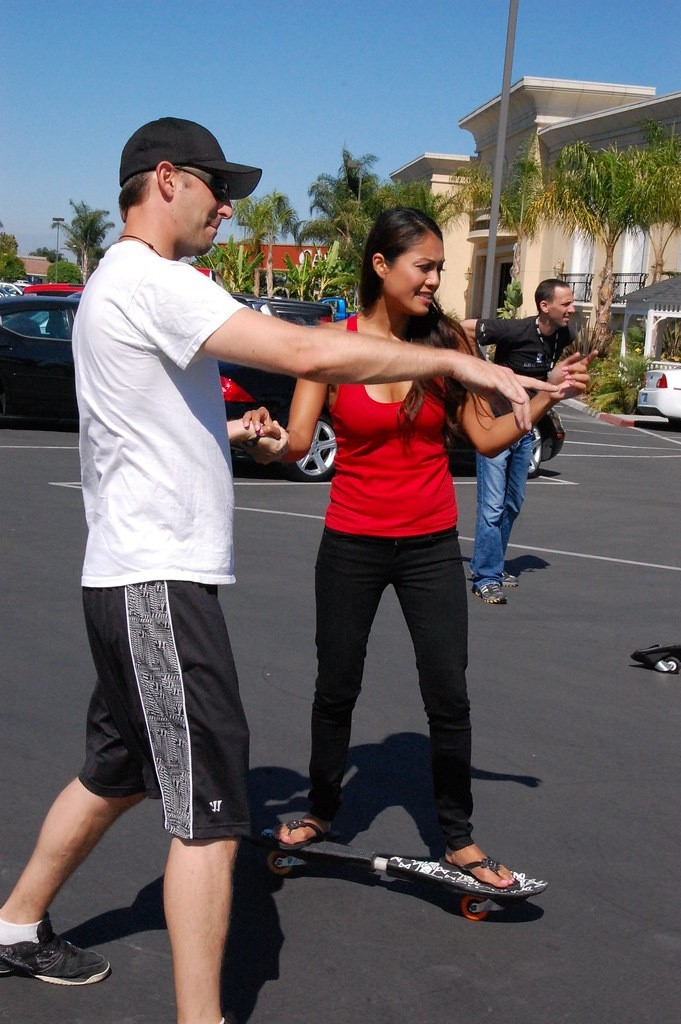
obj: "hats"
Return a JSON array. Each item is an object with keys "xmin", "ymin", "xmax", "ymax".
[{"xmin": 119, "ymin": 117, "xmax": 262, "ymax": 199}]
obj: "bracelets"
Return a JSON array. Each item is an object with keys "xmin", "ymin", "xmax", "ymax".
[{"xmin": 241, "ymin": 435, "xmax": 261, "ymax": 448}]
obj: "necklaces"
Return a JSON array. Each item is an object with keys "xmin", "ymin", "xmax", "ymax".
[
  {"xmin": 535, "ymin": 313, "xmax": 559, "ymax": 378},
  {"xmin": 119, "ymin": 234, "xmax": 163, "ymax": 257}
]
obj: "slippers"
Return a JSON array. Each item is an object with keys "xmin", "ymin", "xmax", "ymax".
[
  {"xmin": 439, "ymin": 855, "xmax": 520, "ymax": 892},
  {"xmin": 274, "ymin": 817, "xmax": 341, "ymax": 850}
]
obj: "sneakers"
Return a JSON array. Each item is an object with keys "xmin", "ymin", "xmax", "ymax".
[
  {"xmin": 467, "ymin": 570, "xmax": 519, "ymax": 587},
  {"xmin": 474, "ymin": 584, "xmax": 507, "ymax": 604},
  {"xmin": 0, "ymin": 911, "xmax": 110, "ymax": 985}
]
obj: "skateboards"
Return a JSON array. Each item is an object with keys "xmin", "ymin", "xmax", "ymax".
[
  {"xmin": 237, "ymin": 813, "xmax": 549, "ymax": 922},
  {"xmin": 630, "ymin": 642, "xmax": 681, "ymax": 674}
]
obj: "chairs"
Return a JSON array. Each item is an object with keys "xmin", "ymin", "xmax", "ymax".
[
  {"xmin": 4, "ymin": 315, "xmax": 41, "ymax": 338},
  {"xmin": 44, "ymin": 310, "xmax": 68, "ymax": 338}
]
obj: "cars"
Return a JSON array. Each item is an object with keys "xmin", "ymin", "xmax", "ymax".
[
  {"xmin": 0, "ymin": 294, "xmax": 80, "ymax": 423},
  {"xmin": 1, "ymin": 281, "xmax": 87, "ymax": 332},
  {"xmin": 215, "ymin": 294, "xmax": 566, "ymax": 482},
  {"xmin": 637, "ymin": 369, "xmax": 681, "ymax": 426}
]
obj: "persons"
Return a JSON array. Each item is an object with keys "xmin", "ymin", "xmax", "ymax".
[
  {"xmin": 457, "ymin": 276, "xmax": 578, "ymax": 605},
  {"xmin": 240, "ymin": 204, "xmax": 602, "ymax": 888},
  {"xmin": 2, "ymin": 113, "xmax": 561, "ymax": 1022}
]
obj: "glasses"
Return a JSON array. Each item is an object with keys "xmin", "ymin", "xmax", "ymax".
[{"xmin": 174, "ymin": 165, "xmax": 230, "ymax": 201}]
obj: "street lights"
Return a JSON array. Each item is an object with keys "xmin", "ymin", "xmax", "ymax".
[
  {"xmin": 348, "ymin": 159, "xmax": 361, "ymax": 204},
  {"xmin": 53, "ymin": 217, "xmax": 66, "ymax": 284}
]
